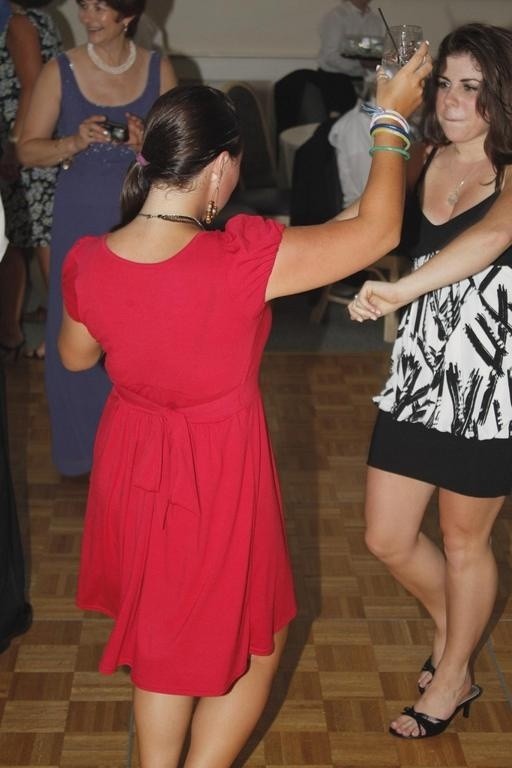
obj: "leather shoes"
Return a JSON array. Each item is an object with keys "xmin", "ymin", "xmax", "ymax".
[{"xmin": 25, "ymin": 350, "xmax": 45, "ymax": 360}]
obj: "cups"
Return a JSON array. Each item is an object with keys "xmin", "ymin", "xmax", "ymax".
[{"xmin": 380, "ymin": 25, "xmax": 423, "ymax": 82}]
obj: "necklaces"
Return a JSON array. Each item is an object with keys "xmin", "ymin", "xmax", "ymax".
[
  {"xmin": 444, "ymin": 160, "xmax": 479, "ymax": 206},
  {"xmin": 137, "ymin": 212, "xmax": 208, "ymax": 234},
  {"xmin": 87, "ymin": 37, "xmax": 137, "ymax": 76}
]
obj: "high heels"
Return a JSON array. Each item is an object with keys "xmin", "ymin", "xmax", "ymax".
[
  {"xmin": 1, "ymin": 338, "xmax": 26, "ymax": 361},
  {"xmin": 389, "ymin": 654, "xmax": 482, "ymax": 739}
]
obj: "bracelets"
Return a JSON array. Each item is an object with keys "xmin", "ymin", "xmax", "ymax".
[{"xmin": 367, "ymin": 106, "xmax": 412, "ymax": 166}]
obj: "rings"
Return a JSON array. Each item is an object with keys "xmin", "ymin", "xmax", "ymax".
[
  {"xmin": 88, "ymin": 131, "xmax": 94, "ymax": 138},
  {"xmin": 354, "ymin": 293, "xmax": 359, "ymax": 300}
]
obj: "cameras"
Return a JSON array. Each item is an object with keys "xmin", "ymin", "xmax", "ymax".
[{"xmin": 103, "ymin": 123, "xmax": 130, "ymax": 144}]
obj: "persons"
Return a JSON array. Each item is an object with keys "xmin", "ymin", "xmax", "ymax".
[
  {"xmin": 14, "ymin": 2, "xmax": 182, "ymax": 481},
  {"xmin": 313, "ymin": 2, "xmax": 388, "ymax": 127},
  {"xmin": 0, "ymin": 96, "xmax": 35, "ymax": 656},
  {"xmin": 323, "ymin": 19, "xmax": 512, "ymax": 742},
  {"xmin": 55, "ymin": 36, "xmax": 438, "ymax": 768},
  {"xmin": 326, "ymin": 55, "xmax": 436, "ymax": 256},
  {"xmin": 1, "ymin": 0, "xmax": 78, "ymax": 365}
]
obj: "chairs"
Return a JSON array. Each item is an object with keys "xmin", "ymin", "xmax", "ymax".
[{"xmin": 226, "ymin": 67, "xmax": 411, "ymax": 342}]
